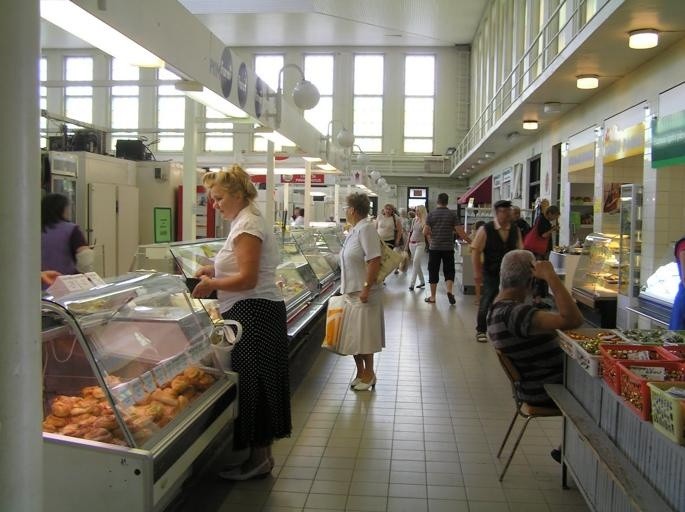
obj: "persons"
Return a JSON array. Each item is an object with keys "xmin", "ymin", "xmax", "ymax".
[
  {"xmin": 291, "ymin": 207, "xmax": 300, "ymax": 221},
  {"xmin": 376, "ymin": 193, "xmax": 560, "ymax": 342},
  {"xmin": 486, "ymin": 249, "xmax": 583, "ymax": 464},
  {"xmin": 191, "ymin": 166, "xmax": 291, "ymax": 480},
  {"xmin": 42, "ymin": 194, "xmax": 88, "ymax": 289},
  {"xmin": 41, "ymin": 270, "xmax": 61, "ymax": 286},
  {"xmin": 294, "ymin": 209, "xmax": 304, "ymax": 225},
  {"xmin": 669, "ymin": 238, "xmax": 685, "ymax": 331},
  {"xmin": 336, "ymin": 193, "xmax": 386, "ymax": 391}
]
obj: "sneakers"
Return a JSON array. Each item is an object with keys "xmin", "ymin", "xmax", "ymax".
[{"xmin": 476, "ymin": 332, "xmax": 488, "ymax": 343}]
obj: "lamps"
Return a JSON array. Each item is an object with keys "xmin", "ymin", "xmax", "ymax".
[
  {"xmin": 543, "ymin": 101, "xmax": 560, "ymax": 115},
  {"xmin": 506, "ymin": 121, "xmax": 538, "ymax": 141},
  {"xmin": 455, "ymin": 153, "xmax": 495, "ymax": 179},
  {"xmin": 628, "ymin": 30, "xmax": 659, "ymax": 49},
  {"xmin": 321, "ymin": 121, "xmax": 391, "ymax": 194},
  {"xmin": 262, "ymin": 64, "xmax": 320, "ymax": 123},
  {"xmin": 577, "ymin": 75, "xmax": 599, "ymax": 90}
]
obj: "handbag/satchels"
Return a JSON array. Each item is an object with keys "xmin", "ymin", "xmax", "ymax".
[
  {"xmin": 320, "ymin": 294, "xmax": 348, "ymax": 356},
  {"xmin": 523, "ymin": 217, "xmax": 551, "ymax": 256},
  {"xmin": 207, "ymin": 319, "xmax": 234, "ymax": 373},
  {"xmin": 358, "ymin": 222, "xmax": 402, "ymax": 286}
]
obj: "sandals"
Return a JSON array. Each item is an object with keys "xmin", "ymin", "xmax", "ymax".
[
  {"xmin": 223, "ymin": 456, "xmax": 275, "ymax": 473},
  {"xmin": 216, "ymin": 458, "xmax": 271, "ymax": 481}
]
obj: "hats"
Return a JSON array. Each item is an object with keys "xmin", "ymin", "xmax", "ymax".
[{"xmin": 494, "ymin": 200, "xmax": 512, "ymax": 208}]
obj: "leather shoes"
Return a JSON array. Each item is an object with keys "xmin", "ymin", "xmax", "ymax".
[
  {"xmin": 394, "ymin": 269, "xmax": 425, "ymax": 290},
  {"xmin": 349, "ymin": 377, "xmax": 362, "ymax": 387}
]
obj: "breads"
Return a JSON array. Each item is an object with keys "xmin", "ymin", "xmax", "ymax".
[{"xmin": 42, "ymin": 366, "xmax": 216, "ymax": 447}]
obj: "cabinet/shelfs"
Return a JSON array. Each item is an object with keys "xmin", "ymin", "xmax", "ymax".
[
  {"xmin": 41, "ymin": 224, "xmax": 346, "ymax": 512},
  {"xmin": 569, "ymin": 184, "xmax": 642, "ymax": 330}
]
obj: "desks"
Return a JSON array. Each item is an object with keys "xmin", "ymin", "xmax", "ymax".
[{"xmin": 545, "ymin": 344, "xmax": 685, "ymax": 512}]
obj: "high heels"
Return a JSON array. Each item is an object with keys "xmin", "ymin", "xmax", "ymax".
[{"xmin": 353, "ymin": 372, "xmax": 377, "ymax": 391}]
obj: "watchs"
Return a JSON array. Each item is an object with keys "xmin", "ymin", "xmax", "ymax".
[{"xmin": 363, "ymin": 281, "xmax": 371, "ymax": 289}]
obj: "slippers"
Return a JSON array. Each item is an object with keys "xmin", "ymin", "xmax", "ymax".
[
  {"xmin": 550, "ymin": 448, "xmax": 561, "ymax": 465},
  {"xmin": 425, "ymin": 296, "xmax": 436, "ymax": 304},
  {"xmin": 447, "ymin": 292, "xmax": 456, "ymax": 304}
]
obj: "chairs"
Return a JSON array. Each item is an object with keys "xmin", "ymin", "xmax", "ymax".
[{"xmin": 495, "ymin": 346, "xmax": 564, "ymax": 482}]
